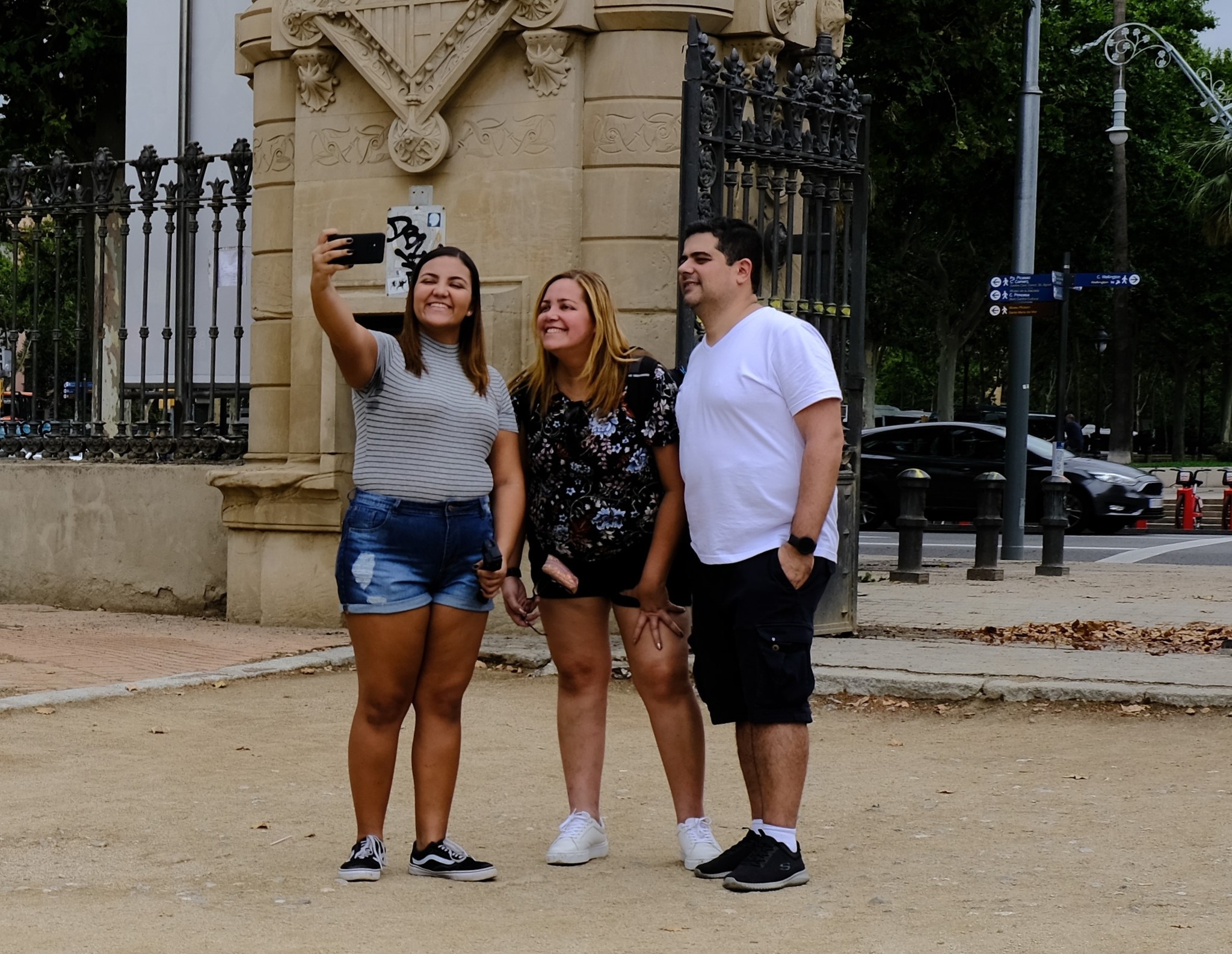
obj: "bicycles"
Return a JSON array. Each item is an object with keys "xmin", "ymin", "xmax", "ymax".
[
  {"xmin": 1169, "ymin": 467, "xmax": 1213, "ymax": 529},
  {"xmin": 1216, "ymin": 467, "xmax": 1232, "ymax": 530}
]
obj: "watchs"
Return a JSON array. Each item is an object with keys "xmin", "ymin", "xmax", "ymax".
[{"xmin": 786, "ymin": 533, "xmax": 817, "ymax": 557}]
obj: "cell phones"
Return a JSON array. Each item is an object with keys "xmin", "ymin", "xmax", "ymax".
[{"xmin": 327, "ymin": 233, "xmax": 385, "ymax": 264}]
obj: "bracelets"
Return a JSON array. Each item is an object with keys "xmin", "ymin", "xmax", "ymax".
[{"xmin": 505, "ymin": 568, "xmax": 522, "ymax": 578}]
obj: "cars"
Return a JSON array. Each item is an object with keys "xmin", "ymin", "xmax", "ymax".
[
  {"xmin": 840, "ymin": 422, "xmax": 1166, "ymax": 536},
  {"xmin": 841, "ymin": 402, "xmax": 1142, "ymax": 456}
]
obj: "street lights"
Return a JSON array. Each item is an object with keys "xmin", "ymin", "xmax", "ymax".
[
  {"xmin": 1090, "ymin": 325, "xmax": 1113, "ymax": 459},
  {"xmin": 1070, "ymin": 21, "xmax": 1232, "ymax": 146}
]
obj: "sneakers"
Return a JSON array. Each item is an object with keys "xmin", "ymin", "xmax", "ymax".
[
  {"xmin": 338, "ymin": 834, "xmax": 387, "ymax": 880},
  {"xmin": 546, "ymin": 808, "xmax": 609, "ymax": 863},
  {"xmin": 408, "ymin": 838, "xmax": 497, "ymax": 881},
  {"xmin": 722, "ymin": 829, "xmax": 810, "ymax": 891},
  {"xmin": 676, "ymin": 816, "xmax": 724, "ymax": 870},
  {"xmin": 694, "ymin": 826, "xmax": 761, "ymax": 879}
]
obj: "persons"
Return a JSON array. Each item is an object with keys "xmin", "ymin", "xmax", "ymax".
[
  {"xmin": 488, "ymin": 268, "xmax": 725, "ymax": 870},
  {"xmin": 1046, "ymin": 413, "xmax": 1083, "ymax": 458},
  {"xmin": 309, "ymin": 227, "xmax": 524, "ymax": 881},
  {"xmin": 672, "ymin": 216, "xmax": 842, "ymax": 891}
]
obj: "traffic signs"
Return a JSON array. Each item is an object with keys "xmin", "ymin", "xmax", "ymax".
[
  {"xmin": 984, "ymin": 304, "xmax": 1055, "ymax": 317},
  {"xmin": 988, "ymin": 287, "xmax": 1052, "ymax": 302},
  {"xmin": 1053, "ymin": 286, "xmax": 1063, "ymax": 301},
  {"xmin": 1051, "ymin": 270, "xmax": 1065, "ymax": 286},
  {"xmin": 1074, "ymin": 273, "xmax": 1143, "ymax": 287},
  {"xmin": 990, "ymin": 273, "xmax": 1052, "ymax": 289}
]
